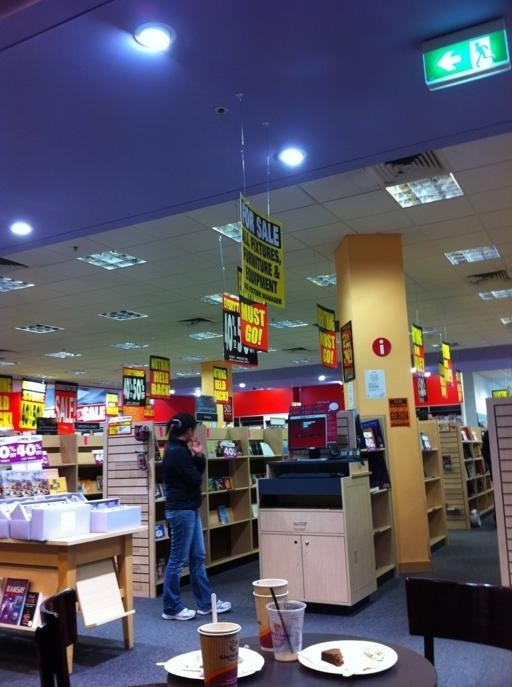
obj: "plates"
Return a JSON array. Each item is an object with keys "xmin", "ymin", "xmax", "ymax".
[
  {"xmin": 297, "ymin": 639, "xmax": 399, "ymax": 677},
  {"xmin": 162, "ymin": 647, "xmax": 268, "ymax": 680}
]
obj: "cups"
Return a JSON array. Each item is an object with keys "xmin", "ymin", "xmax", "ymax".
[
  {"xmin": 267, "ymin": 600, "xmax": 307, "ymax": 663},
  {"xmin": 195, "ymin": 622, "xmax": 241, "ymax": 687},
  {"xmin": 252, "ymin": 578, "xmax": 289, "ymax": 651}
]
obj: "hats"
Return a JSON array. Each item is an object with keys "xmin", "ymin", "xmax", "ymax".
[{"xmin": 171, "ymin": 413, "xmax": 201, "ymax": 433}]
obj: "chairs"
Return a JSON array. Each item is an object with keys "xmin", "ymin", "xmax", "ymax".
[{"xmin": 401, "ymin": 575, "xmax": 509, "ymax": 685}]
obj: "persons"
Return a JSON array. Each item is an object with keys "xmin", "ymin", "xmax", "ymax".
[{"xmin": 159, "ymin": 412, "xmax": 232, "ymax": 621}]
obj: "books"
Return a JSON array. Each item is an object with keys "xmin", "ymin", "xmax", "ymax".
[
  {"xmin": 155, "ymin": 440, "xmax": 287, "ymax": 537},
  {"xmin": 0, "ymin": 469, "xmax": 119, "ymax": 510},
  {"xmin": 20, "ymin": 592, "xmax": 38, "ymax": 628},
  {"xmin": 419, "ymin": 423, "xmax": 492, "ymax": 515},
  {"xmin": 1, "ymin": 576, "xmax": 30, "ymax": 624}
]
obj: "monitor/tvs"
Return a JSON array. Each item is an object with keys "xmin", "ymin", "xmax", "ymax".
[{"xmin": 288, "ymin": 415, "xmax": 328, "ymax": 461}]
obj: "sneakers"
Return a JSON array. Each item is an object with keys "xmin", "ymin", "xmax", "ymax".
[
  {"xmin": 162, "ymin": 607, "xmax": 196, "ymax": 622},
  {"xmin": 197, "ymin": 600, "xmax": 232, "ymax": 615}
]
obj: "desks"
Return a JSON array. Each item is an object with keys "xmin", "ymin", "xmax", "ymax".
[
  {"xmin": 166, "ymin": 634, "xmax": 440, "ymax": 686},
  {"xmin": 1, "ymin": 521, "xmax": 150, "ymax": 677}
]
